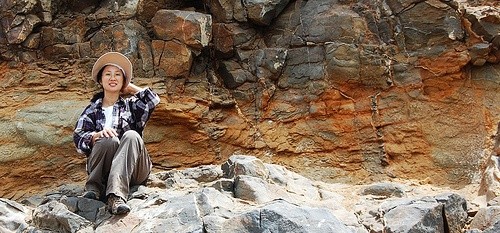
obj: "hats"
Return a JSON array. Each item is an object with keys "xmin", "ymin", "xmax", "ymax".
[{"xmin": 90, "ymin": 51, "xmax": 132, "ymax": 90}]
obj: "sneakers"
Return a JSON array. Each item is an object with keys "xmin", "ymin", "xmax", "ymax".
[
  {"xmin": 108, "ymin": 195, "xmax": 130, "ymax": 216},
  {"xmin": 82, "ymin": 190, "xmax": 99, "ymax": 201}
]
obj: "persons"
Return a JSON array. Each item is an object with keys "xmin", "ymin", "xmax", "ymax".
[{"xmin": 72, "ymin": 52, "xmax": 161, "ymax": 214}]
